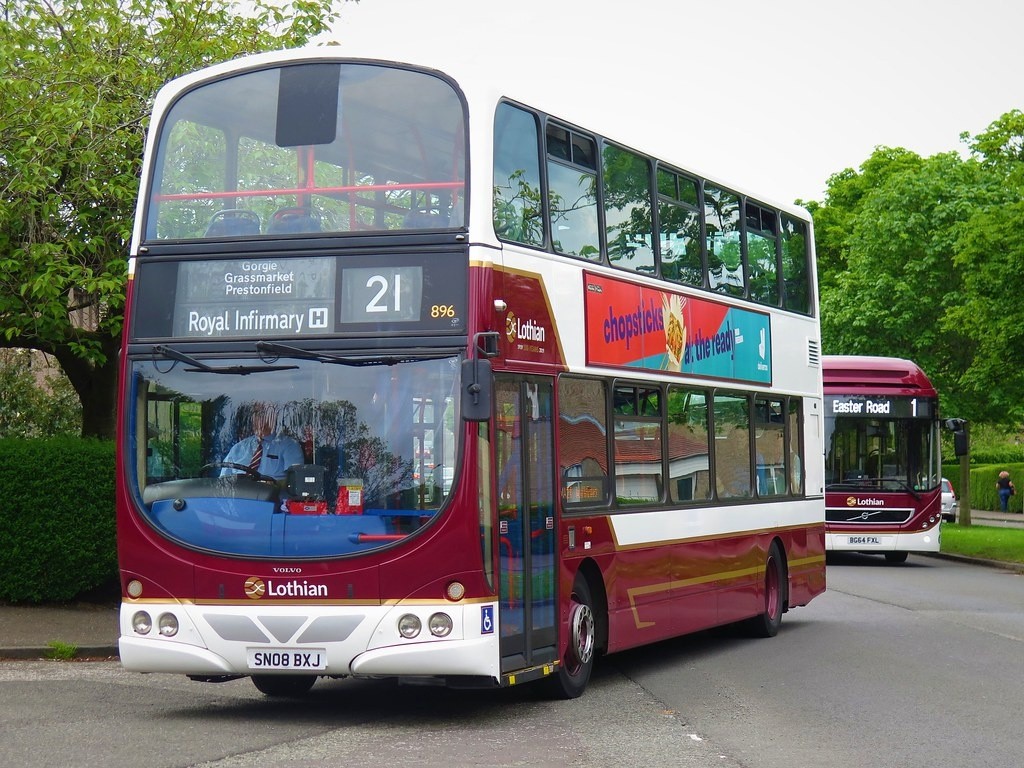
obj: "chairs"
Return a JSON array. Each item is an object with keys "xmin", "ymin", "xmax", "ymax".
[
  {"xmin": 400, "ymin": 205, "xmax": 452, "ymax": 228},
  {"xmin": 201, "ymin": 207, "xmax": 322, "ymax": 239},
  {"xmin": 869, "ymin": 448, "xmax": 886, "ymax": 482}
]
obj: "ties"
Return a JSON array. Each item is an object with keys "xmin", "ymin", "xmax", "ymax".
[{"xmin": 246, "ymin": 439, "xmax": 264, "ymax": 477}]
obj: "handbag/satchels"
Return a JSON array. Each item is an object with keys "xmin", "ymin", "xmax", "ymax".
[{"xmin": 1011, "ymin": 488, "xmax": 1016, "ymax": 494}]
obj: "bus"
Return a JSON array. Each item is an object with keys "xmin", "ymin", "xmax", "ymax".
[
  {"xmin": 113, "ymin": 43, "xmax": 830, "ymax": 702},
  {"xmin": 821, "ymin": 353, "xmax": 969, "ymax": 564}
]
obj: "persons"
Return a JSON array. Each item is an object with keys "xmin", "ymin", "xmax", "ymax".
[
  {"xmin": 717, "ymin": 426, "xmax": 802, "ymax": 498},
  {"xmin": 995, "ymin": 471, "xmax": 1014, "ymax": 513},
  {"xmin": 220, "ymin": 390, "xmax": 304, "ymax": 501}
]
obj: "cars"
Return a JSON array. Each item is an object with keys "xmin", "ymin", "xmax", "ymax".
[{"xmin": 920, "ymin": 476, "xmax": 957, "ymax": 523}]
